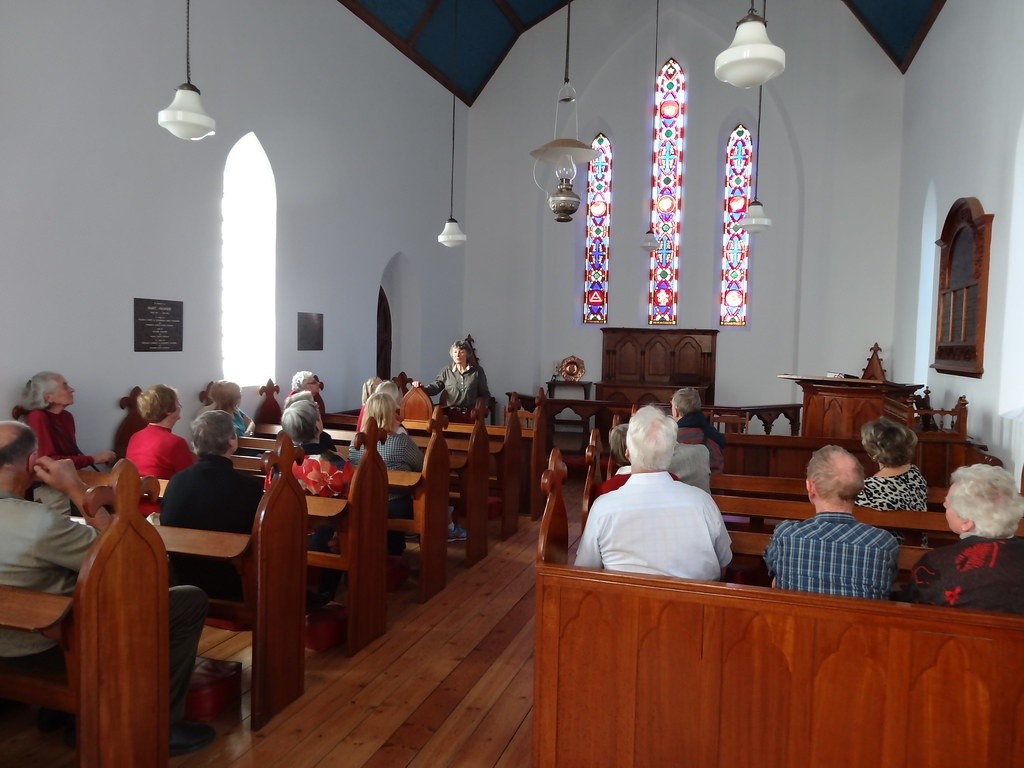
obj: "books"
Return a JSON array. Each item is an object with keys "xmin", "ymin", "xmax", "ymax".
[{"xmin": 826, "ymin": 371, "xmax": 859, "ymax": 379}]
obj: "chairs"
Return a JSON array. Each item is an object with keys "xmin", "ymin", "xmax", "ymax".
[{"xmin": 710, "ymin": 409, "xmax": 749, "ymax": 434}]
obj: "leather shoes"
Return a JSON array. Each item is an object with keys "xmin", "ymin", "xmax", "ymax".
[{"xmin": 167, "ymin": 720, "xmax": 216, "ymax": 756}]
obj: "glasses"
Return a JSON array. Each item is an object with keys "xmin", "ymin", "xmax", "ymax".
[{"xmin": 307, "ymin": 379, "xmax": 317, "ymax": 385}]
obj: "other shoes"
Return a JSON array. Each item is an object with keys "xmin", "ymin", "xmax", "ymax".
[{"xmin": 307, "ymin": 584, "xmax": 335, "ymax": 614}]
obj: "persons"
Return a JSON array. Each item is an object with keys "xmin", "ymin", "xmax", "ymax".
[
  {"xmin": 412, "ymin": 340, "xmax": 489, "ymax": 424},
  {"xmin": 0, "ymin": 419, "xmax": 216, "ymax": 756},
  {"xmin": 854, "ymin": 417, "xmax": 927, "ymax": 548},
  {"xmin": 763, "ymin": 445, "xmax": 900, "ymax": 600},
  {"xmin": 608, "ymin": 393, "xmax": 711, "ymax": 496},
  {"xmin": 573, "ymin": 405, "xmax": 733, "ymax": 581},
  {"xmin": 288, "ymin": 371, "xmax": 320, "ymax": 396},
  {"xmin": 903, "ymin": 464, "xmax": 1024, "ymax": 618},
  {"xmin": 22, "ymin": 372, "xmax": 117, "ymax": 516},
  {"xmin": 270, "ymin": 392, "xmax": 406, "ymax": 556},
  {"xmin": 670, "ymin": 387, "xmax": 726, "ymax": 474},
  {"xmin": 348, "ymin": 393, "xmax": 466, "ymax": 542},
  {"xmin": 125, "ymin": 384, "xmax": 194, "ymax": 519},
  {"xmin": 159, "ymin": 410, "xmax": 339, "ymax": 609},
  {"xmin": 197, "ymin": 381, "xmax": 266, "ymax": 458},
  {"xmin": 356, "ymin": 377, "xmax": 408, "ymax": 435}
]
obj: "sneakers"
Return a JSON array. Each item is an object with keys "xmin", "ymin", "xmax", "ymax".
[{"xmin": 447, "ymin": 524, "xmax": 468, "ymax": 542}]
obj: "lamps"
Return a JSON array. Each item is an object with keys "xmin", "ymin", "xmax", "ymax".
[
  {"xmin": 438, "ymin": 0, "xmax": 466, "ymax": 246},
  {"xmin": 530, "ymin": 0, "xmax": 602, "ymax": 222},
  {"xmin": 714, "ymin": 0, "xmax": 786, "ymax": 89},
  {"xmin": 739, "ymin": 0, "xmax": 771, "ymax": 234},
  {"xmin": 158, "ymin": 0, "xmax": 216, "ymax": 141},
  {"xmin": 640, "ymin": 0, "xmax": 660, "ymax": 251}
]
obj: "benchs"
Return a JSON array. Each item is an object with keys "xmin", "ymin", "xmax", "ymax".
[
  {"xmin": 1, "ymin": 380, "xmax": 521, "ymax": 767},
  {"xmin": 530, "ymin": 415, "xmax": 1023, "ymax": 768}
]
